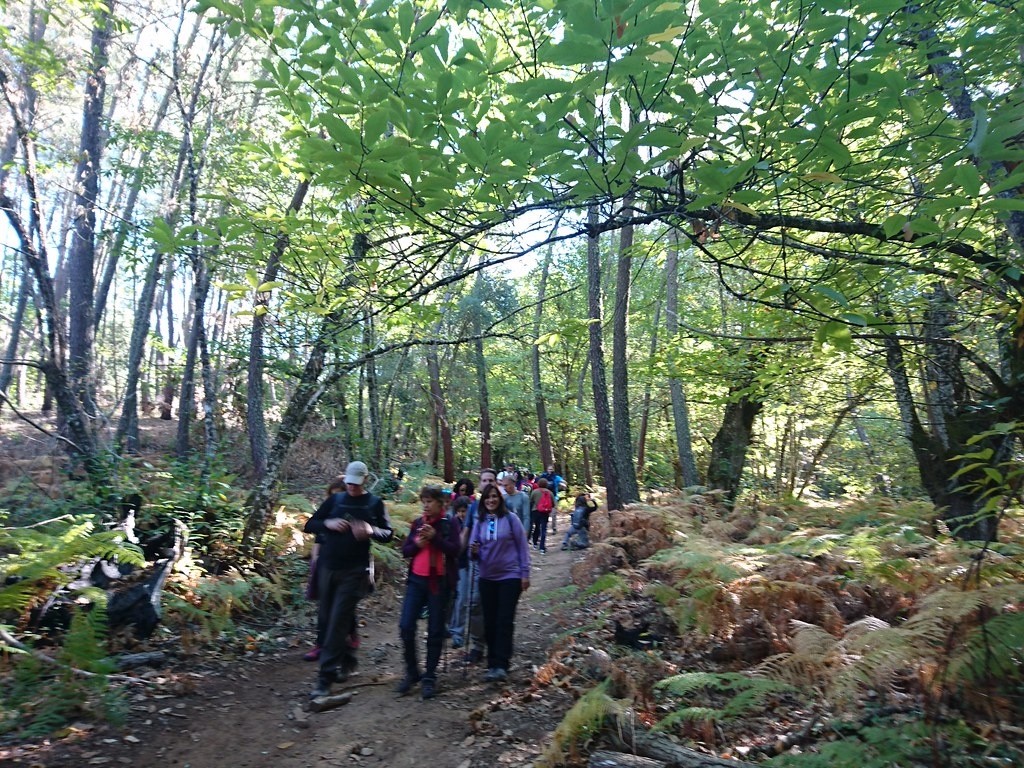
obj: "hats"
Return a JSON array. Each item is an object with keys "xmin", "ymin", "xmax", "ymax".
[{"xmin": 343, "ymin": 460, "xmax": 370, "ymax": 486}]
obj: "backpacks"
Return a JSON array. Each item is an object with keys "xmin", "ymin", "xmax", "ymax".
[
  {"xmin": 572, "ymin": 507, "xmax": 589, "ymax": 529},
  {"xmin": 521, "ymin": 483, "xmax": 533, "ymax": 497},
  {"xmin": 537, "ymin": 488, "xmax": 552, "ymax": 513}
]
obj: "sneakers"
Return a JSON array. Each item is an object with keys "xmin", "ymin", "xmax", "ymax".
[
  {"xmin": 346, "ymin": 635, "xmax": 360, "ymax": 649},
  {"xmin": 334, "ymin": 661, "xmax": 350, "ymax": 683},
  {"xmin": 310, "ymin": 676, "xmax": 332, "ymax": 700},
  {"xmin": 396, "ymin": 673, "xmax": 422, "ymax": 692},
  {"xmin": 480, "ymin": 668, "xmax": 507, "ymax": 682},
  {"xmin": 420, "ymin": 674, "xmax": 436, "ymax": 699},
  {"xmin": 303, "ymin": 646, "xmax": 323, "ymax": 660}
]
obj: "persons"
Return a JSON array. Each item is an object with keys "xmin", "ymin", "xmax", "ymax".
[
  {"xmin": 539, "ymin": 464, "xmax": 567, "ymax": 535},
  {"xmin": 465, "ymin": 484, "xmax": 531, "ymax": 682},
  {"xmin": 456, "ymin": 467, "xmax": 517, "ymax": 661},
  {"xmin": 529, "ymin": 477, "xmax": 557, "ymax": 554},
  {"xmin": 394, "ymin": 484, "xmax": 461, "ymax": 699},
  {"xmin": 301, "ymin": 461, "xmax": 392, "ymax": 700},
  {"xmin": 303, "ymin": 484, "xmax": 375, "ymax": 661},
  {"xmin": 561, "ymin": 493, "xmax": 597, "ymax": 550},
  {"xmin": 419, "ymin": 462, "xmax": 539, "ymax": 620}
]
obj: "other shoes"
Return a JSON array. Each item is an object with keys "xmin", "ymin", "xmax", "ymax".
[
  {"xmin": 444, "ymin": 629, "xmax": 452, "ymax": 637},
  {"xmin": 553, "ymin": 529, "xmax": 557, "ymax": 533},
  {"xmin": 540, "ymin": 548, "xmax": 546, "ymax": 555},
  {"xmin": 462, "ymin": 649, "xmax": 483, "ymax": 663},
  {"xmin": 561, "ymin": 545, "xmax": 568, "ymax": 550},
  {"xmin": 532, "ymin": 545, "xmax": 537, "ymax": 551},
  {"xmin": 454, "ymin": 638, "xmax": 464, "ymax": 648},
  {"xmin": 528, "ymin": 540, "xmax": 532, "ymax": 545},
  {"xmin": 570, "ymin": 541, "xmax": 575, "ymax": 550}
]
